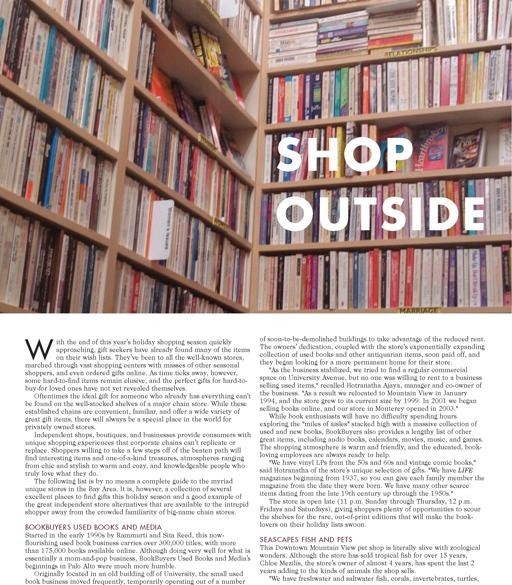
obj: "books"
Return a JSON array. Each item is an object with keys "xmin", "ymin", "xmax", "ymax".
[
  {"xmin": 270, "ymin": 0, "xmax": 512, "ymax": 65},
  {"xmin": 260, "ymin": 245, "xmax": 512, "ymax": 310},
  {"xmin": 0, "ymin": 207, "xmax": 107, "ymax": 313},
  {"xmin": 261, "ymin": 180, "xmax": 453, "ymax": 244},
  {"xmin": 0, "ymin": 95, "xmax": 115, "ymax": 240},
  {"xmin": 403, "ymin": 48, "xmax": 512, "ymax": 110},
  {"xmin": 267, "ymin": 62, "xmax": 403, "ymax": 122},
  {"xmin": 380, "ymin": 126, "xmax": 410, "ymax": 174},
  {"xmin": 147, "ymin": 0, "xmax": 259, "ymax": 121},
  {"xmin": 455, "ymin": 176, "xmax": 512, "ymax": 235},
  {"xmin": 452, "ymin": 128, "xmax": 486, "ymax": 168},
  {"xmin": 204, "ymin": 0, "xmax": 263, "ymax": 67},
  {"xmin": 140, "ymin": 24, "xmax": 254, "ymax": 176},
  {"xmin": 39, "ymin": 0, "xmax": 132, "ymax": 70},
  {"xmin": 265, "ymin": 122, "xmax": 380, "ymax": 181},
  {"xmin": 115, "ymin": 260, "xmax": 237, "ymax": 312},
  {"xmin": 411, "ymin": 123, "xmax": 449, "ymax": 170},
  {"xmin": 0, "ymin": 0, "xmax": 123, "ymax": 150},
  {"xmin": 498, "ymin": 124, "xmax": 512, "ymax": 165},
  {"xmin": 129, "ymin": 95, "xmax": 253, "ymax": 238},
  {"xmin": 121, "ymin": 176, "xmax": 249, "ymax": 306}
]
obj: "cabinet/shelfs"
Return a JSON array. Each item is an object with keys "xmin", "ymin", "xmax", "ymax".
[
  {"xmin": 252, "ymin": 0, "xmax": 511, "ymax": 314},
  {"xmin": 0, "ymin": 0, "xmax": 265, "ymax": 315}
]
